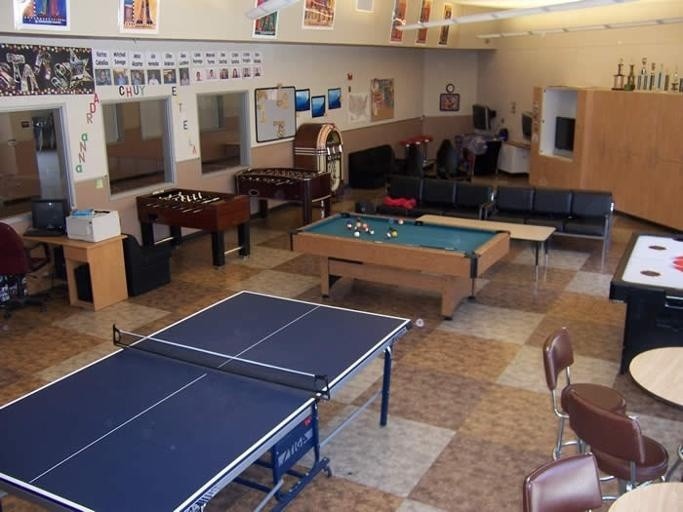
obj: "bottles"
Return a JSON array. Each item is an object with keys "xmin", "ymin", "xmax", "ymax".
[{"xmin": 612, "ymin": 56, "xmax": 682, "ymax": 94}]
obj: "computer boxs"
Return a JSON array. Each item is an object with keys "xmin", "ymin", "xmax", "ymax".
[{"xmin": 75, "ymin": 264, "xmax": 93, "ymax": 302}]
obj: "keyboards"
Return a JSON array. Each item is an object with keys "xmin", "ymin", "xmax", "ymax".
[{"xmin": 23, "ymin": 230, "xmax": 62, "ymax": 236}]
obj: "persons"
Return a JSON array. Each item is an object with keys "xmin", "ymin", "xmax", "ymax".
[
  {"xmin": 180, "ymin": 71, "xmax": 189, "ymax": 85},
  {"xmin": 243, "ymin": 68, "xmax": 250, "ymax": 77},
  {"xmin": 221, "ymin": 69, "xmax": 228, "ymax": 79},
  {"xmin": 149, "ymin": 73, "xmax": 159, "ymax": 84},
  {"xmin": 208, "ymin": 70, "xmax": 216, "ymax": 79},
  {"xmin": 232, "ymin": 68, "xmax": 239, "ymax": 78},
  {"xmin": 254, "ymin": 67, "xmax": 261, "ymax": 76},
  {"xmin": 116, "ymin": 72, "xmax": 127, "ymax": 85},
  {"xmin": 132, "ymin": 72, "xmax": 142, "ymax": 85},
  {"xmin": 97, "ymin": 70, "xmax": 111, "ymax": 85},
  {"xmin": 165, "ymin": 72, "xmax": 174, "ymax": 83},
  {"xmin": 195, "ymin": 72, "xmax": 202, "ymax": 81}
]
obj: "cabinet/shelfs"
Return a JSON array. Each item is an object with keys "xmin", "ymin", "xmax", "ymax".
[{"xmin": 525, "ymin": 82, "xmax": 682, "ymax": 232}]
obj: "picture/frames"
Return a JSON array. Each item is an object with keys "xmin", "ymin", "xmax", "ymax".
[{"xmin": 439, "ymin": 93, "xmax": 459, "ymax": 111}]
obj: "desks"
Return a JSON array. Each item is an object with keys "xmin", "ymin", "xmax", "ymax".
[
  {"xmin": 607, "ymin": 229, "xmax": 682, "ymax": 368},
  {"xmin": 600, "ymin": 477, "xmax": 683, "ymax": 512},
  {"xmin": 630, "ymin": 347, "xmax": 682, "ymax": 477},
  {"xmin": 0, "ymin": 294, "xmax": 407, "ymax": 510},
  {"xmin": 20, "ymin": 226, "xmax": 127, "ymax": 311},
  {"xmin": 286, "ymin": 207, "xmax": 510, "ymax": 318},
  {"xmin": 137, "ymin": 183, "xmax": 248, "ymax": 268},
  {"xmin": 230, "ymin": 160, "xmax": 330, "ymax": 225}
]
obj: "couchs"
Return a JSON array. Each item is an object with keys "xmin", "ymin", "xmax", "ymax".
[{"xmin": 347, "ymin": 142, "xmax": 402, "ymax": 188}]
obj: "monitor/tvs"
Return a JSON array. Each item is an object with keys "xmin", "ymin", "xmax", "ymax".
[
  {"xmin": 521, "ymin": 112, "xmax": 532, "ymax": 141},
  {"xmin": 32, "ymin": 199, "xmax": 68, "ymax": 229},
  {"xmin": 553, "ymin": 116, "xmax": 575, "ymax": 157},
  {"xmin": 472, "ymin": 104, "xmax": 496, "ymax": 136}
]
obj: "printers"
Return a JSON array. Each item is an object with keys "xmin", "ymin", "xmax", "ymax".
[{"xmin": 65, "ymin": 209, "xmax": 122, "ymax": 242}]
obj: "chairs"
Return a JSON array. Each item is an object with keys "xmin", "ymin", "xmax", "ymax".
[
  {"xmin": 118, "ymin": 231, "xmax": 170, "ymax": 294},
  {"xmin": 563, "ymin": 392, "xmax": 670, "ymax": 500},
  {"xmin": 0, "ymin": 221, "xmax": 44, "ymax": 320},
  {"xmin": 433, "ymin": 138, "xmax": 471, "ymax": 183},
  {"xmin": 540, "ymin": 326, "xmax": 621, "ymax": 458},
  {"xmin": 519, "ymin": 449, "xmax": 601, "ymax": 512}
]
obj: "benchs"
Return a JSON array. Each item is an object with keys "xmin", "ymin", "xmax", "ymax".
[
  {"xmin": 482, "ymin": 182, "xmax": 613, "ymax": 268},
  {"xmin": 372, "ymin": 175, "xmax": 494, "ymax": 220}
]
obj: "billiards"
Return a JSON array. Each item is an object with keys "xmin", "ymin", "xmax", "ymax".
[
  {"xmin": 415, "ymin": 318, "xmax": 425, "ymax": 327},
  {"xmin": 347, "ymin": 220, "xmax": 352, "ymax": 230},
  {"xmin": 353, "ymin": 231, "xmax": 360, "ymax": 237},
  {"xmin": 355, "ymin": 217, "xmax": 374, "ymax": 235},
  {"xmin": 384, "ymin": 218, "xmax": 398, "ymax": 240},
  {"xmin": 397, "ymin": 218, "xmax": 403, "ymax": 225}
]
